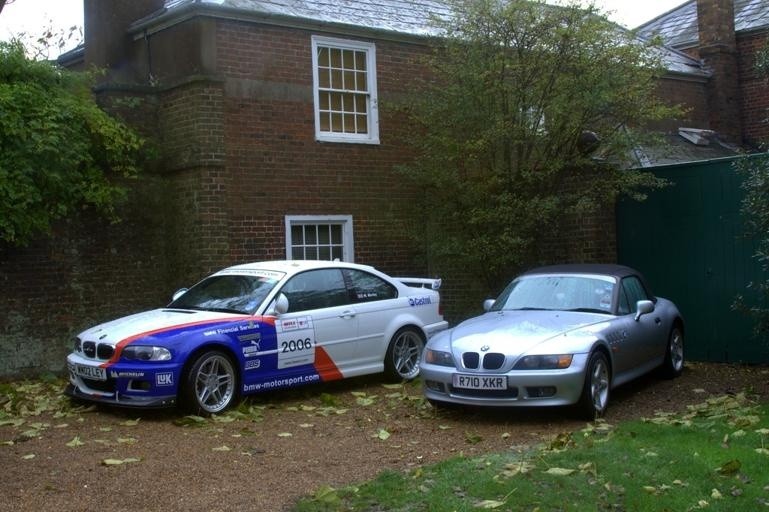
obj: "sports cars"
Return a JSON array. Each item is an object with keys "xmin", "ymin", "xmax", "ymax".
[{"xmin": 419, "ymin": 263, "xmax": 687, "ymax": 420}]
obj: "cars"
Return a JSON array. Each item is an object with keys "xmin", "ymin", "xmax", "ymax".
[{"xmin": 61, "ymin": 257, "xmax": 449, "ymax": 417}]
